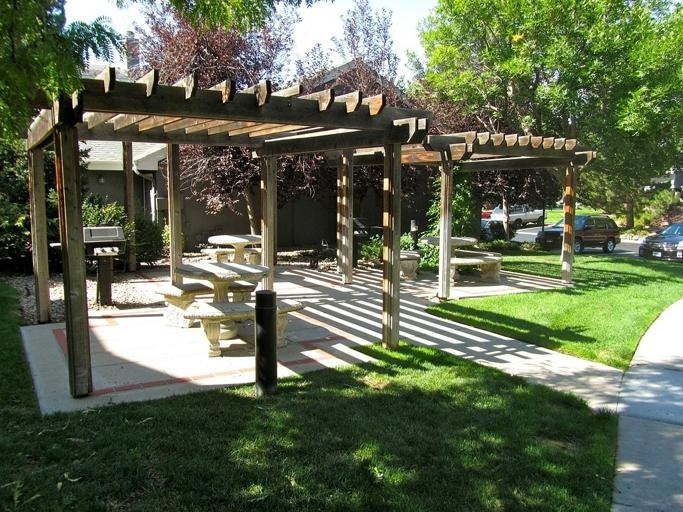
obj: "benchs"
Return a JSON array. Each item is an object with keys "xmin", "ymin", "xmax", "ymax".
[
  {"xmin": 448, "ymin": 253, "xmax": 503, "ymax": 285},
  {"xmin": 200, "ymin": 247, "xmax": 262, "ymax": 264},
  {"xmin": 181, "ymin": 298, "xmax": 307, "ymax": 358},
  {"xmin": 155, "ymin": 280, "xmax": 257, "ymax": 328},
  {"xmin": 455, "ymin": 249, "xmax": 502, "ymax": 271},
  {"xmin": 400, "ymin": 249, "xmax": 420, "ymax": 280}
]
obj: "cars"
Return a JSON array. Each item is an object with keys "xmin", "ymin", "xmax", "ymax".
[
  {"xmin": 639, "ymin": 223, "xmax": 683, "ymax": 262},
  {"xmin": 480, "ymin": 202, "xmax": 544, "ymax": 241}
]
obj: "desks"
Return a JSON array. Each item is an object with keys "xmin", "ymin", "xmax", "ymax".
[
  {"xmin": 418, "ymin": 236, "xmax": 479, "ymax": 280},
  {"xmin": 175, "ymin": 261, "xmax": 270, "ymax": 339},
  {"xmin": 207, "ymin": 234, "xmax": 263, "ymax": 263}
]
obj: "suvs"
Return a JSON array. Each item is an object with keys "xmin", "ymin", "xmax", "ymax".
[{"xmin": 536, "ymin": 215, "xmax": 621, "ymax": 254}]
